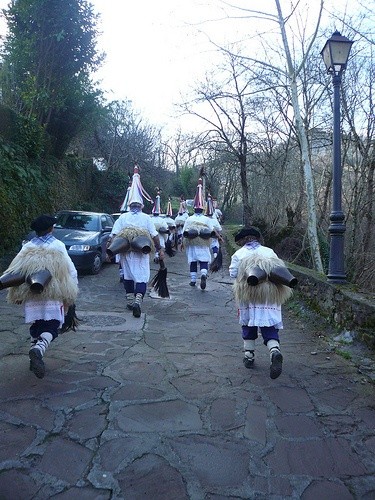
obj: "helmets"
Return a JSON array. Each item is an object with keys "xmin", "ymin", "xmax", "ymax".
[
  {"xmin": 166, "ymin": 193, "xmax": 173, "ymax": 216},
  {"xmin": 178, "ymin": 196, "xmax": 187, "ymax": 215},
  {"xmin": 208, "ymin": 194, "xmax": 218, "ymax": 208},
  {"xmin": 119, "ymin": 173, "xmax": 132, "ymax": 212},
  {"xmin": 204, "ymin": 196, "xmax": 213, "ymax": 216},
  {"xmin": 152, "ymin": 186, "xmax": 162, "ymax": 214},
  {"xmin": 194, "ymin": 178, "xmax": 206, "ymax": 210},
  {"xmin": 127, "ymin": 162, "xmax": 154, "ymax": 206}
]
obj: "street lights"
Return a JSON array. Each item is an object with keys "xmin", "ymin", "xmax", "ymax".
[{"xmin": 318, "ymin": 27, "xmax": 356, "ymax": 283}]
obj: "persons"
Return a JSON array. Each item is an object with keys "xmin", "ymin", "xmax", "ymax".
[
  {"xmin": 116, "ymin": 209, "xmax": 222, "ymax": 282},
  {"xmin": 182, "ymin": 207, "xmax": 213, "ymax": 290},
  {"xmin": 107, "ymin": 202, "xmax": 165, "ymax": 318},
  {"xmin": 229, "ymin": 225, "xmax": 284, "ymax": 379},
  {"xmin": 8, "ymin": 213, "xmax": 78, "ymax": 379}
]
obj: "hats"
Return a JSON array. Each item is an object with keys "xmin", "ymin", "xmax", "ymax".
[
  {"xmin": 31, "ymin": 214, "xmax": 57, "ymax": 230},
  {"xmin": 234, "ymin": 225, "xmax": 264, "ymax": 245}
]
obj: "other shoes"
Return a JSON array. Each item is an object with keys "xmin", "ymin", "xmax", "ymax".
[
  {"xmin": 268, "ymin": 346, "xmax": 283, "ymax": 379},
  {"xmin": 200, "ymin": 273, "xmax": 207, "ymax": 290},
  {"xmin": 132, "ymin": 293, "xmax": 143, "ymax": 318},
  {"xmin": 153, "ymin": 253, "xmax": 160, "ymax": 264},
  {"xmin": 126, "ymin": 293, "xmax": 134, "ymax": 309},
  {"xmin": 189, "ymin": 280, "xmax": 196, "ymax": 286},
  {"xmin": 243, "ymin": 349, "xmax": 255, "ymax": 367},
  {"xmin": 28, "ymin": 345, "xmax": 45, "ymax": 379}
]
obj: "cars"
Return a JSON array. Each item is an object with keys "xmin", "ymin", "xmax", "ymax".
[{"xmin": 18, "ymin": 209, "xmax": 117, "ymax": 276}]
